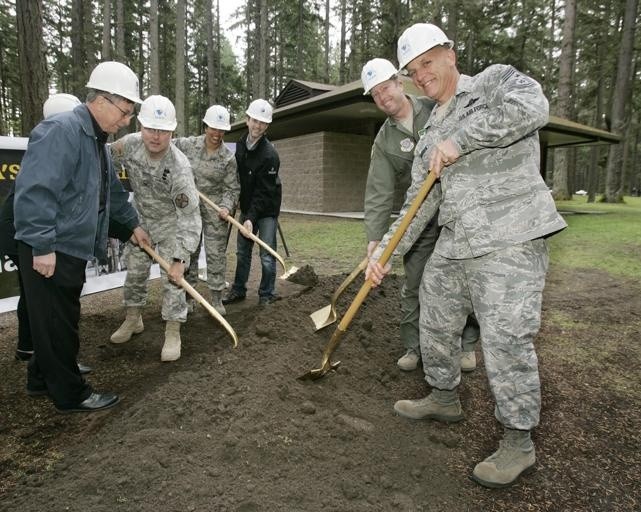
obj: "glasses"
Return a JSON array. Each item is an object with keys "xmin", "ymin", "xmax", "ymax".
[{"xmin": 103, "ymin": 95, "xmax": 135, "ymax": 120}]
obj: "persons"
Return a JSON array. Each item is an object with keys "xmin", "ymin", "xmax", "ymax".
[
  {"xmin": 223, "ymin": 97, "xmax": 283, "ymax": 306},
  {"xmin": 0, "ymin": 92, "xmax": 93, "ymax": 375},
  {"xmin": 10, "ymin": 58, "xmax": 153, "ymax": 415},
  {"xmin": 173, "ymin": 103, "xmax": 243, "ymax": 318},
  {"xmin": 360, "ymin": 56, "xmax": 481, "ymax": 374},
  {"xmin": 390, "ymin": 21, "xmax": 570, "ymax": 491},
  {"xmin": 106, "ymin": 93, "xmax": 204, "ymax": 364}
]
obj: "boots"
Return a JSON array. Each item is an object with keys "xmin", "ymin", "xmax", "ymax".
[
  {"xmin": 471, "ymin": 424, "xmax": 538, "ymax": 489},
  {"xmin": 109, "ymin": 305, "xmax": 145, "ymax": 344},
  {"xmin": 210, "ymin": 289, "xmax": 227, "ymax": 317},
  {"xmin": 393, "ymin": 388, "xmax": 465, "ymax": 424},
  {"xmin": 160, "ymin": 319, "xmax": 182, "ymax": 362}
]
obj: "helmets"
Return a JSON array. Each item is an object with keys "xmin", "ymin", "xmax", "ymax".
[
  {"xmin": 395, "ymin": 21, "xmax": 455, "ymax": 75},
  {"xmin": 84, "ymin": 61, "xmax": 147, "ymax": 104},
  {"xmin": 42, "ymin": 92, "xmax": 82, "ymax": 120},
  {"xmin": 136, "ymin": 94, "xmax": 177, "ymax": 132},
  {"xmin": 202, "ymin": 105, "xmax": 232, "ymax": 132},
  {"xmin": 360, "ymin": 58, "xmax": 398, "ymax": 96},
  {"xmin": 245, "ymin": 98, "xmax": 273, "ymax": 124}
]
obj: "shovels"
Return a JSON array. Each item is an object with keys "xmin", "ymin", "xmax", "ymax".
[
  {"xmin": 193, "ymin": 190, "xmax": 310, "ymax": 285},
  {"xmin": 295, "ymin": 160, "xmax": 444, "ymax": 381},
  {"xmin": 309, "ymin": 241, "xmax": 378, "ymax": 331}
]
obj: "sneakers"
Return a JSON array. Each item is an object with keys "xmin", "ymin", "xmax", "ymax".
[
  {"xmin": 222, "ymin": 285, "xmax": 247, "ymax": 305},
  {"xmin": 258, "ymin": 292, "xmax": 282, "ymax": 305},
  {"xmin": 395, "ymin": 349, "xmax": 420, "ymax": 372},
  {"xmin": 459, "ymin": 350, "xmax": 477, "ymax": 373}
]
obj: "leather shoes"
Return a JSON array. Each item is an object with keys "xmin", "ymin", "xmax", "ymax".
[
  {"xmin": 15, "ymin": 349, "xmax": 35, "ymax": 365},
  {"xmin": 76, "ymin": 362, "xmax": 92, "ymax": 374},
  {"xmin": 25, "ymin": 381, "xmax": 45, "ymax": 396},
  {"xmin": 54, "ymin": 390, "xmax": 120, "ymax": 415}
]
